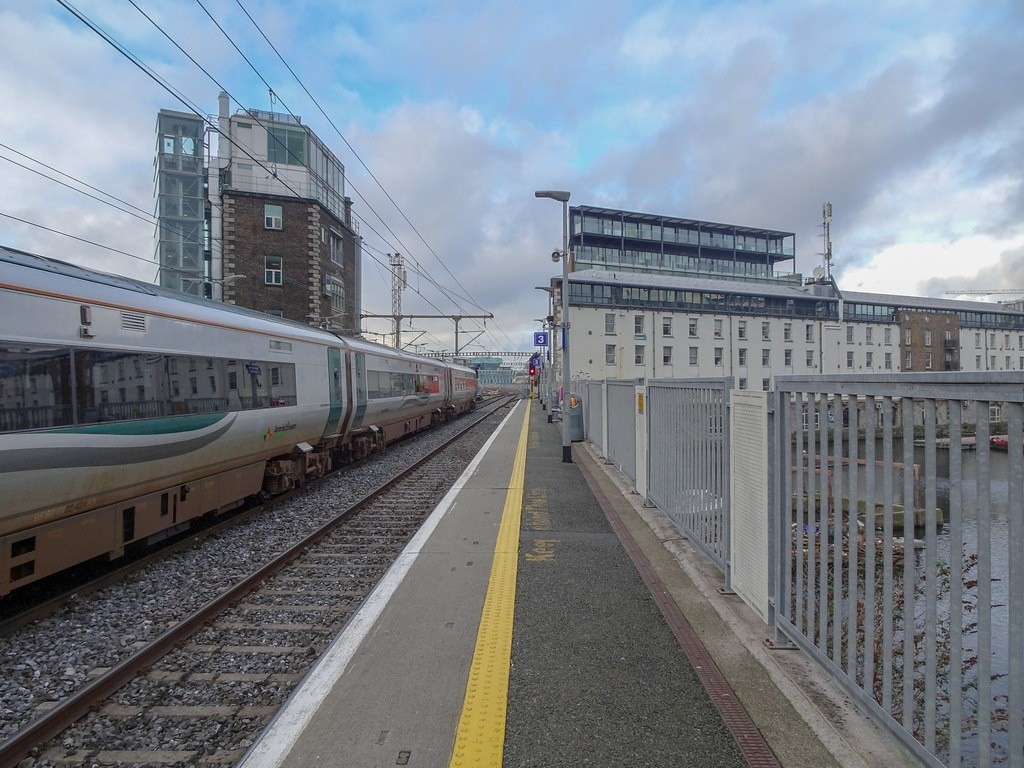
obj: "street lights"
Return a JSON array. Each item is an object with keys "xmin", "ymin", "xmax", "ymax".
[
  {"xmin": 535, "ymin": 286, "xmax": 553, "ymax": 423},
  {"xmin": 306, "ymin": 313, "xmax": 348, "ymax": 330},
  {"xmin": 534, "ymin": 318, "xmax": 546, "ymax": 409},
  {"xmin": 181, "ymin": 274, "xmax": 246, "ymax": 300},
  {"xmin": 404, "ymin": 342, "xmax": 428, "ymax": 354},
  {"xmin": 535, "ymin": 190, "xmax": 572, "ymax": 463},
  {"xmin": 369, "ymin": 331, "xmax": 397, "ymax": 345}
]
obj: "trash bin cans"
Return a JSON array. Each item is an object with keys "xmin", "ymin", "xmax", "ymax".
[
  {"xmin": 559, "ymin": 392, "xmax": 584, "ymax": 443},
  {"xmin": 548, "ymin": 389, "xmax": 561, "ymax": 412}
]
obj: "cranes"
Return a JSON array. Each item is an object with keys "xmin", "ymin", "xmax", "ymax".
[{"xmin": 944, "ymin": 288, "xmax": 1023, "ymax": 294}]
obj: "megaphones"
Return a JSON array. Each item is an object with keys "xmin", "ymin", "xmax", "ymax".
[{"xmin": 551, "ymin": 252, "xmax": 561, "ymax": 262}]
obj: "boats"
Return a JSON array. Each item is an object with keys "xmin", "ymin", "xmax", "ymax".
[{"xmin": 669, "ymin": 489, "xmax": 723, "ymax": 521}]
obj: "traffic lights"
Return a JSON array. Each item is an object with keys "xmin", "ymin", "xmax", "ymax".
[
  {"xmin": 529, "ymin": 363, "xmax": 535, "ymax": 375},
  {"xmin": 532, "ymin": 380, "xmax": 538, "ymax": 386}
]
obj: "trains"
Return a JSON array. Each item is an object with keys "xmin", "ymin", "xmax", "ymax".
[{"xmin": 0, "ymin": 245, "xmax": 475, "ymax": 596}]
